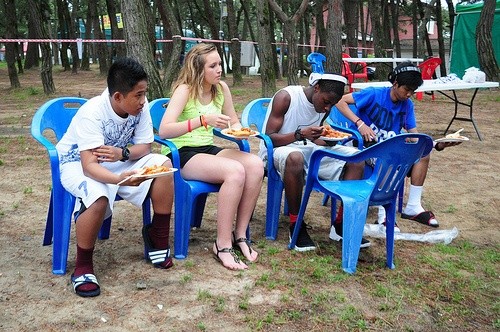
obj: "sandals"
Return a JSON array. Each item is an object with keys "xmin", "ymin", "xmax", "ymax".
[
  {"xmin": 212, "ymin": 240, "xmax": 250, "ymax": 272},
  {"xmin": 231, "ymin": 231, "xmax": 260, "ymax": 263}
]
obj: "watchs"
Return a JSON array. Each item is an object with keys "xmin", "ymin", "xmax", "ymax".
[
  {"xmin": 119, "ymin": 146, "xmax": 130, "ymax": 163},
  {"xmin": 294, "ymin": 129, "xmax": 307, "ymax": 145}
]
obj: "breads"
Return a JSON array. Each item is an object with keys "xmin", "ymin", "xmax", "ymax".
[{"xmin": 232, "ymin": 131, "xmax": 249, "ymax": 136}]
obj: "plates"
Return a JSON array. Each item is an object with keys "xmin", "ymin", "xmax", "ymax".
[
  {"xmin": 319, "ymin": 132, "xmax": 352, "ymax": 142},
  {"xmin": 132, "ymin": 167, "xmax": 178, "ymax": 180},
  {"xmin": 434, "ymin": 132, "xmax": 470, "ymax": 143},
  {"xmin": 220, "ymin": 127, "xmax": 260, "ymax": 140}
]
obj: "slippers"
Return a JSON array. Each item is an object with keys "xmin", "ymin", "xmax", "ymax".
[
  {"xmin": 141, "ymin": 224, "xmax": 173, "ymax": 268},
  {"xmin": 374, "ymin": 218, "xmax": 401, "ymax": 232},
  {"xmin": 401, "ymin": 210, "xmax": 440, "ymax": 227},
  {"xmin": 71, "ymin": 271, "xmax": 102, "ymax": 297}
]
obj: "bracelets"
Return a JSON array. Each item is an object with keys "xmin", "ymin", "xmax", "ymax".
[
  {"xmin": 357, "ymin": 122, "xmax": 365, "ymax": 130},
  {"xmin": 355, "ymin": 118, "xmax": 361, "ymax": 124},
  {"xmin": 199, "ymin": 115, "xmax": 207, "ymax": 129},
  {"xmin": 187, "ymin": 119, "xmax": 192, "ymax": 132},
  {"xmin": 435, "ymin": 142, "xmax": 444, "ymax": 151}
]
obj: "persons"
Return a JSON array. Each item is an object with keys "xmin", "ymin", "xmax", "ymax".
[
  {"xmin": 55, "ymin": 55, "xmax": 175, "ymax": 297},
  {"xmin": 336, "ymin": 62, "xmax": 462, "ymax": 233},
  {"xmin": 158, "ymin": 43, "xmax": 265, "ymax": 272},
  {"xmin": 259, "ymin": 71, "xmax": 370, "ymax": 252}
]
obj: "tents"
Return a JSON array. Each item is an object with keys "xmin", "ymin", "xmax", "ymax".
[{"xmin": 448, "ymin": 0, "xmax": 500, "ymax": 89}]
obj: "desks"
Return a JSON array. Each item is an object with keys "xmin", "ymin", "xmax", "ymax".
[
  {"xmin": 350, "ymin": 77, "xmax": 500, "ymax": 142},
  {"xmin": 341, "ymin": 57, "xmax": 424, "ymax": 107}
]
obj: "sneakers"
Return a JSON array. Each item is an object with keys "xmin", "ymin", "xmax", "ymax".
[
  {"xmin": 288, "ymin": 219, "xmax": 316, "ymax": 252},
  {"xmin": 329, "ymin": 221, "xmax": 372, "ymax": 248}
]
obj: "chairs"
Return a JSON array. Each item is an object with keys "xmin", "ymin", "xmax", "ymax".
[
  {"xmin": 307, "ymin": 52, "xmax": 326, "ymax": 87},
  {"xmin": 322, "ymin": 103, "xmax": 404, "ymax": 214},
  {"xmin": 287, "ymin": 132, "xmax": 435, "ymax": 275},
  {"xmin": 147, "ymin": 98, "xmax": 251, "ymax": 259},
  {"xmin": 341, "ymin": 52, "xmax": 368, "ymax": 94},
  {"xmin": 416, "ymin": 56, "xmax": 443, "ymax": 101},
  {"xmin": 31, "ymin": 95, "xmax": 153, "ymax": 275},
  {"xmin": 240, "ymin": 98, "xmax": 337, "ymax": 242}
]
